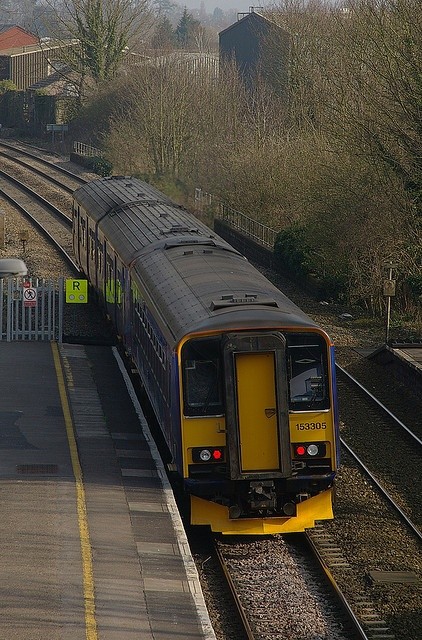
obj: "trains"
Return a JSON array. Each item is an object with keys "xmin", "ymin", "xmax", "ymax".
[{"xmin": 72, "ymin": 174, "xmax": 338, "ymax": 536}]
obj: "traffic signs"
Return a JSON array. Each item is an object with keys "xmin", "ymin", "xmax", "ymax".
[{"xmin": 65, "ymin": 279, "xmax": 88, "ymax": 304}]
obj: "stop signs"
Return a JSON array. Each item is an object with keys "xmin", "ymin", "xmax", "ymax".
[
  {"xmin": 383, "ymin": 280, "xmax": 396, "ymax": 296},
  {"xmin": 22, "ymin": 287, "xmax": 37, "ymax": 308}
]
